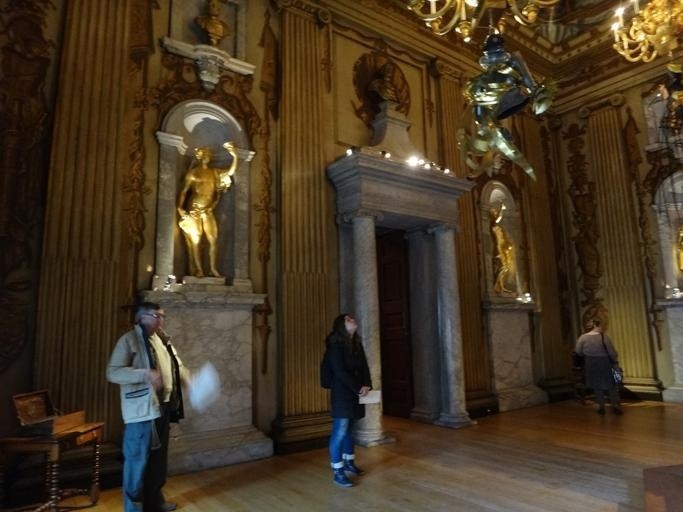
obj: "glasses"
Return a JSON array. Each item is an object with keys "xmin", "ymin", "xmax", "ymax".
[{"xmin": 141, "ymin": 312, "xmax": 167, "ymax": 321}]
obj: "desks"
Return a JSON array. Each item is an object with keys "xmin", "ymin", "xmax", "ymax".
[{"xmin": 2, "ymin": 422, "xmax": 106, "ymax": 512}]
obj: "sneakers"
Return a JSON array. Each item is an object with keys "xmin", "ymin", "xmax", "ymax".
[{"xmin": 156, "ymin": 502, "xmax": 177, "ymax": 511}]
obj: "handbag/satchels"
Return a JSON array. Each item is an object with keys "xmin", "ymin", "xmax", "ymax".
[{"xmin": 609, "ymin": 365, "xmax": 624, "ymax": 385}]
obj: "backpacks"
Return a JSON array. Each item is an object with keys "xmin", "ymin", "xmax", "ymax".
[{"xmin": 319, "ymin": 337, "xmax": 335, "ymax": 392}]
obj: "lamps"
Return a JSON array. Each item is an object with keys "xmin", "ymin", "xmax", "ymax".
[
  {"xmin": 404, "ymin": 1, "xmax": 542, "ymax": 48},
  {"xmin": 404, "ymin": 155, "xmax": 454, "ymax": 175},
  {"xmin": 347, "ymin": 148, "xmax": 353, "ymax": 156},
  {"xmin": 609, "ymin": 2, "xmax": 683, "ymax": 66},
  {"xmin": 381, "ymin": 151, "xmax": 390, "ymax": 159}
]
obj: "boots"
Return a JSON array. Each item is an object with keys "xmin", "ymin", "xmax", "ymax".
[
  {"xmin": 329, "ymin": 460, "xmax": 355, "ymax": 487},
  {"xmin": 340, "ymin": 451, "xmax": 365, "ymax": 475}
]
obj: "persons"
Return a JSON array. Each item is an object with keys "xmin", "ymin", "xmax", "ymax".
[
  {"xmin": 367, "ymin": 62, "xmax": 402, "ymax": 110},
  {"xmin": 193, "ymin": 0, "xmax": 232, "ymax": 46},
  {"xmin": 104, "ymin": 302, "xmax": 194, "ymax": 511},
  {"xmin": 490, "ymin": 200, "xmax": 514, "ymax": 292},
  {"xmin": 574, "ymin": 317, "xmax": 624, "ymax": 414},
  {"xmin": 176, "ymin": 139, "xmax": 239, "ymax": 277},
  {"xmin": 319, "ymin": 313, "xmax": 372, "ymax": 486}
]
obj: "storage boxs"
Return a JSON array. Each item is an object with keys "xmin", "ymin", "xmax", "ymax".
[{"xmin": 14, "ymin": 382, "xmax": 86, "ymax": 435}]
